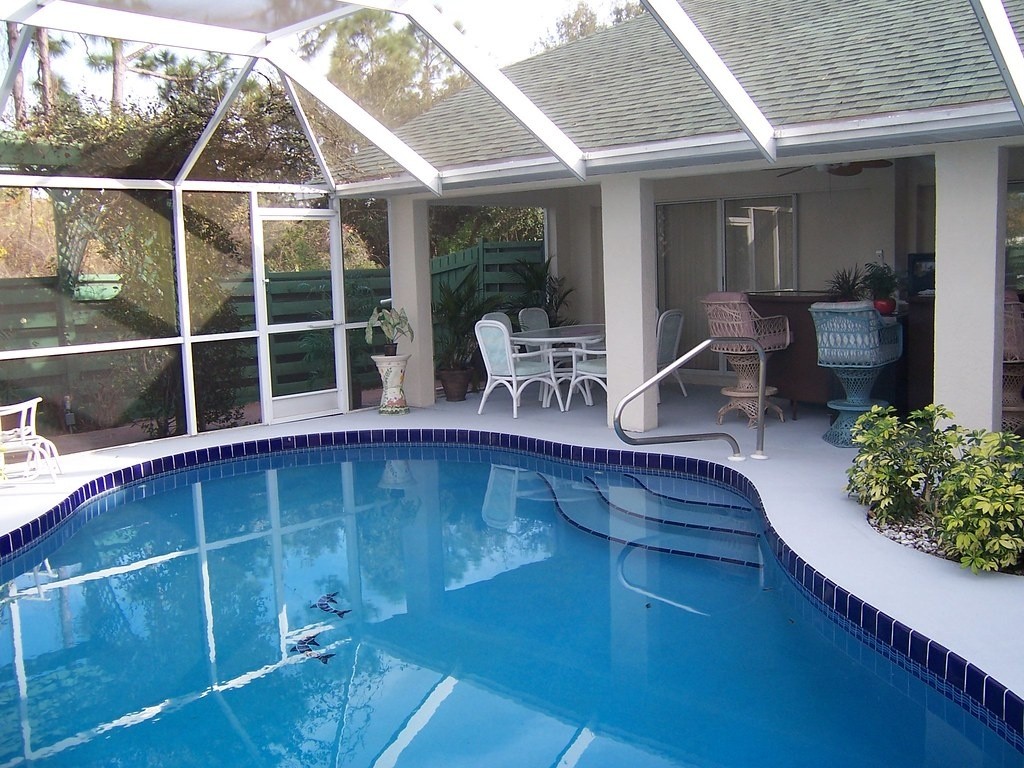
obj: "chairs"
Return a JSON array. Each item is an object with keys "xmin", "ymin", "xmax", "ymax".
[
  {"xmin": 657, "ymin": 309, "xmax": 688, "ymax": 404},
  {"xmin": 0, "ymin": 397, "xmax": 63, "ymax": 479},
  {"xmin": 565, "ymin": 348, "xmax": 607, "ymax": 414},
  {"xmin": 475, "ymin": 307, "xmax": 565, "ymax": 419},
  {"xmin": 1001, "ymin": 302, "xmax": 1024, "ymax": 436},
  {"xmin": 808, "ymin": 301, "xmax": 903, "ymax": 448},
  {"xmin": 700, "ymin": 292, "xmax": 790, "ymax": 429}
]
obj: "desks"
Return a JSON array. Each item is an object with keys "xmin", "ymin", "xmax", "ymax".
[{"xmin": 510, "ymin": 324, "xmax": 605, "ymax": 408}]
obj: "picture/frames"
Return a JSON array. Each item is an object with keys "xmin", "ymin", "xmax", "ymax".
[{"xmin": 907, "ymin": 253, "xmax": 936, "ymax": 297}]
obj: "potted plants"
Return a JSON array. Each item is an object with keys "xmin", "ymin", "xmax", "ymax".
[
  {"xmin": 433, "ymin": 270, "xmax": 510, "ymax": 402},
  {"xmin": 297, "ymin": 280, "xmax": 377, "ymax": 410},
  {"xmin": 365, "ymin": 307, "xmax": 414, "ymax": 415},
  {"xmin": 861, "ymin": 262, "xmax": 901, "ymax": 317}
]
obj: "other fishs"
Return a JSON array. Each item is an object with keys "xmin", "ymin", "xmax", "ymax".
[
  {"xmin": 289, "ymin": 630, "xmax": 336, "ymax": 665},
  {"xmin": 310, "ymin": 591, "xmax": 352, "ymax": 618}
]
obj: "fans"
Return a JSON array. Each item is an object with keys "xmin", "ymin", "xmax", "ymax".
[{"xmin": 777, "ymin": 159, "xmax": 893, "ymax": 178}]
